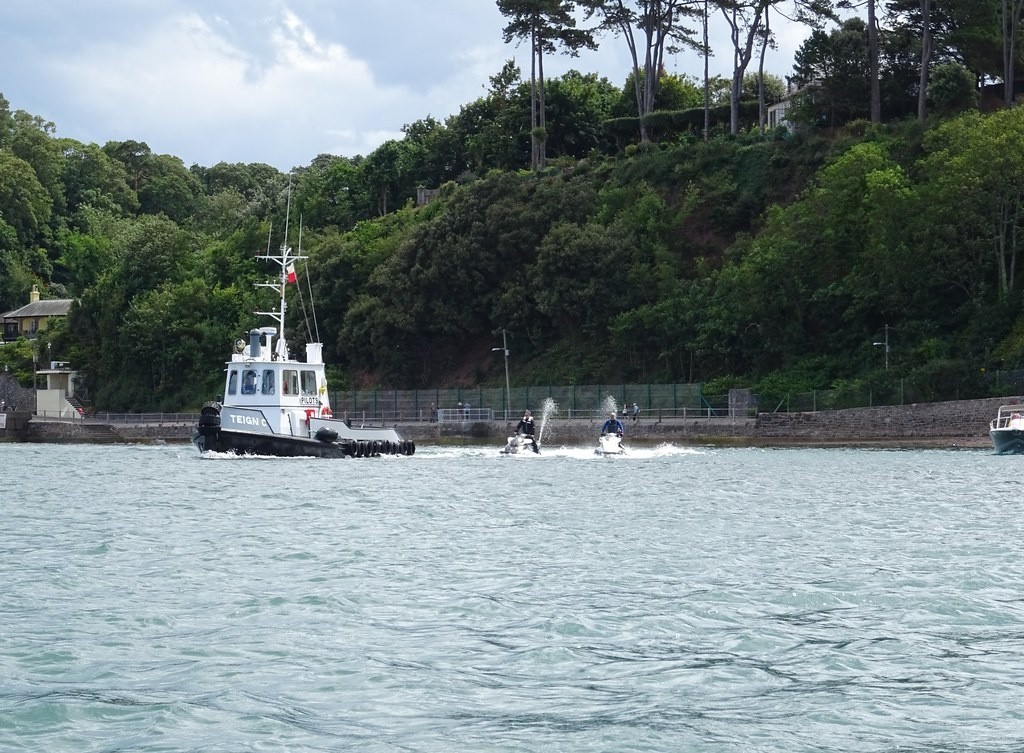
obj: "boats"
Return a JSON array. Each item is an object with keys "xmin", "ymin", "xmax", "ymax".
[
  {"xmin": 190, "ymin": 181, "xmax": 414, "ymax": 461},
  {"xmin": 989, "ymin": 403, "xmax": 1024, "ymax": 458}
]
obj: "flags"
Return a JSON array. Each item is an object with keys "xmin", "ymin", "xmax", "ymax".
[{"xmin": 286, "ymin": 262, "xmax": 296, "ymax": 282}]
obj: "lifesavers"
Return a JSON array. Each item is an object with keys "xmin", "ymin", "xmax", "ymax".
[{"xmin": 321, "ymin": 406, "xmax": 333, "ymax": 419}]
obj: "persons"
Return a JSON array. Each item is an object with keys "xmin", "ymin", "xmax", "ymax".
[
  {"xmin": 431, "ymin": 399, "xmax": 472, "ymax": 422},
  {"xmin": 513, "ymin": 410, "xmax": 537, "ymax": 454},
  {"xmin": 601, "ymin": 413, "xmax": 623, "ymax": 437},
  {"xmin": 622, "ymin": 402, "xmax": 641, "ymax": 421}
]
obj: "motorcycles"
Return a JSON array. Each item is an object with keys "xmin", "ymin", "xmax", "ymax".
[
  {"xmin": 594, "ymin": 432, "xmax": 626, "ymax": 456},
  {"xmin": 500, "ymin": 430, "xmax": 543, "ymax": 457}
]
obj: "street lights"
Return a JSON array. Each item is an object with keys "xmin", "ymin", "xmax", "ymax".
[
  {"xmin": 872, "ymin": 323, "xmax": 890, "ymax": 371},
  {"xmin": 492, "ymin": 327, "xmax": 511, "ymax": 418}
]
